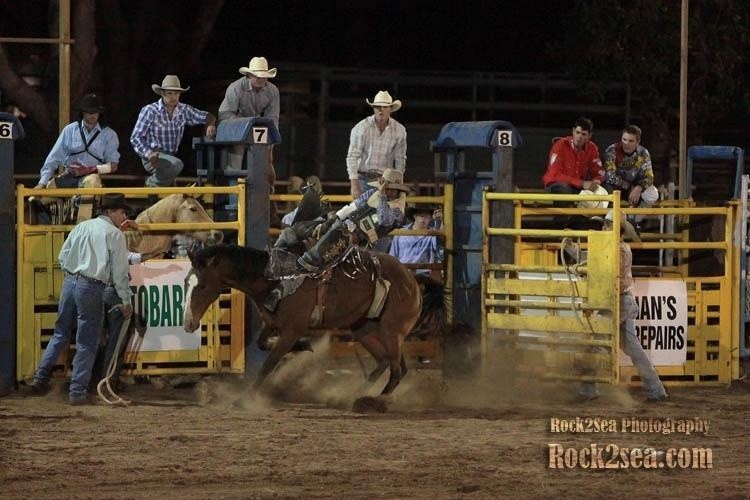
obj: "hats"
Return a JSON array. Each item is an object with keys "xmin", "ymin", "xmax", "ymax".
[
  {"xmin": 367, "ymin": 168, "xmax": 412, "ymax": 196},
  {"xmin": 577, "ymin": 184, "xmax": 610, "ymax": 218},
  {"xmin": 239, "ymin": 56, "xmax": 277, "ymax": 79},
  {"xmin": 79, "ymin": 94, "xmax": 106, "ymax": 114},
  {"xmin": 151, "ymin": 75, "xmax": 191, "ymax": 95},
  {"xmin": 96, "ymin": 193, "xmax": 132, "ymax": 213},
  {"xmin": 365, "ymin": 90, "xmax": 402, "ymax": 112},
  {"xmin": 590, "ymin": 210, "xmax": 640, "ymax": 241},
  {"xmin": 404, "ymin": 203, "xmax": 435, "ymax": 222}
]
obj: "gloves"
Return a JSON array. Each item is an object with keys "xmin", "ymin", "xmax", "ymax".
[{"xmin": 67, "ymin": 161, "xmax": 98, "ymax": 177}]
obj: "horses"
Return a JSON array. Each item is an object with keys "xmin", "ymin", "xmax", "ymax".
[
  {"xmin": 122, "ymin": 192, "xmax": 226, "ymax": 261},
  {"xmin": 180, "ymin": 240, "xmax": 453, "ymax": 417}
]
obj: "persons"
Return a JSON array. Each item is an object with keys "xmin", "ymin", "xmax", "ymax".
[
  {"xmin": 276, "ymin": 167, "xmax": 407, "ymax": 273},
  {"xmin": 345, "ymin": 90, "xmax": 408, "ymax": 198},
  {"xmin": 558, "ymin": 210, "xmax": 671, "ymax": 406},
  {"xmin": 28, "ymin": 93, "xmax": 121, "ymax": 206},
  {"xmin": 216, "ymin": 57, "xmax": 282, "ymax": 221},
  {"xmin": 543, "ymin": 117, "xmax": 606, "ymax": 240},
  {"xmin": 266, "ymin": 176, "xmax": 448, "ymax": 366},
  {"xmin": 62, "ymin": 219, "xmax": 165, "ymax": 396},
  {"xmin": 601, "ymin": 124, "xmax": 659, "ymax": 244},
  {"xmin": 20, "ymin": 192, "xmax": 132, "ymax": 406},
  {"xmin": 128, "ymin": 75, "xmax": 216, "ymax": 187}
]
